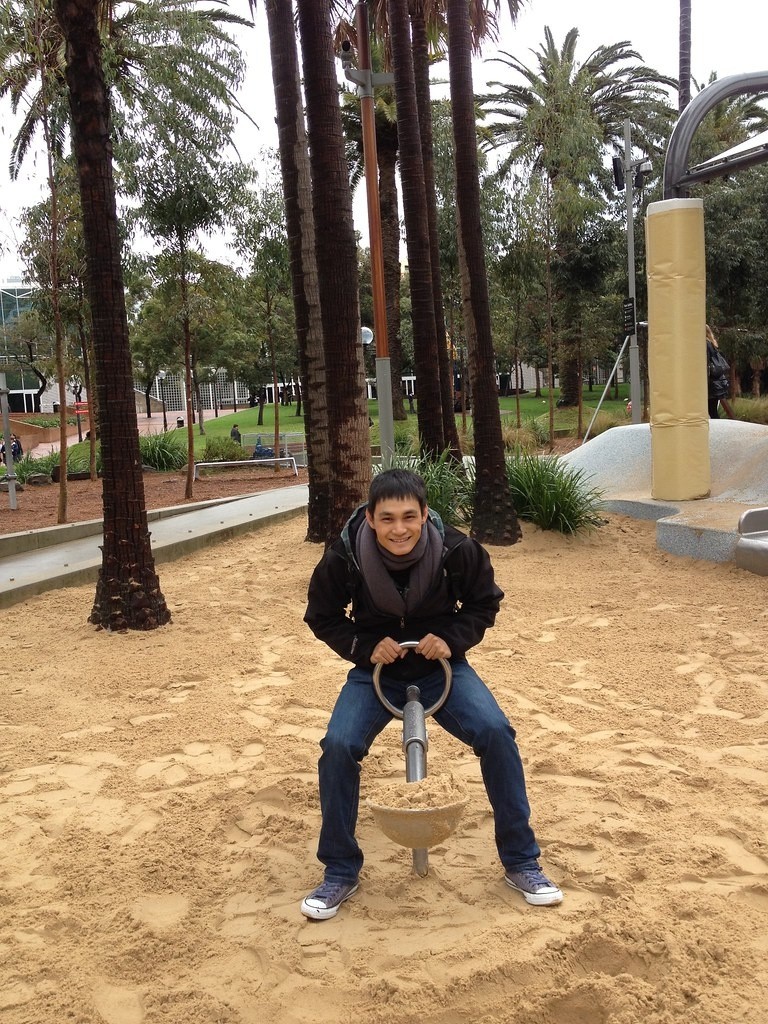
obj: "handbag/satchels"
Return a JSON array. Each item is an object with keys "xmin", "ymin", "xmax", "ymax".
[{"xmin": 709, "ymin": 352, "xmax": 730, "ymax": 380}]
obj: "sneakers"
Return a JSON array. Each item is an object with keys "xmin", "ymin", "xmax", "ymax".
[
  {"xmin": 300, "ymin": 879, "xmax": 358, "ymax": 919},
  {"xmin": 504, "ymin": 861, "xmax": 563, "ymax": 905}
]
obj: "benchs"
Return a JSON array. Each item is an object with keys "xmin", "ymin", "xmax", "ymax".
[{"xmin": 193, "ymin": 457, "xmax": 298, "ymax": 482}]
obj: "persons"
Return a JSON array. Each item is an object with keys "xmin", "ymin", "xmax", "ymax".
[
  {"xmin": 230, "ymin": 424, "xmax": 241, "ymax": 447},
  {"xmin": 706, "ymin": 324, "xmax": 731, "ymax": 419},
  {"xmin": 623, "ymin": 397, "xmax": 632, "ymax": 417},
  {"xmin": 301, "ymin": 468, "xmax": 564, "ymax": 920},
  {"xmin": 0, "ymin": 433, "xmax": 25, "ymax": 468}
]
